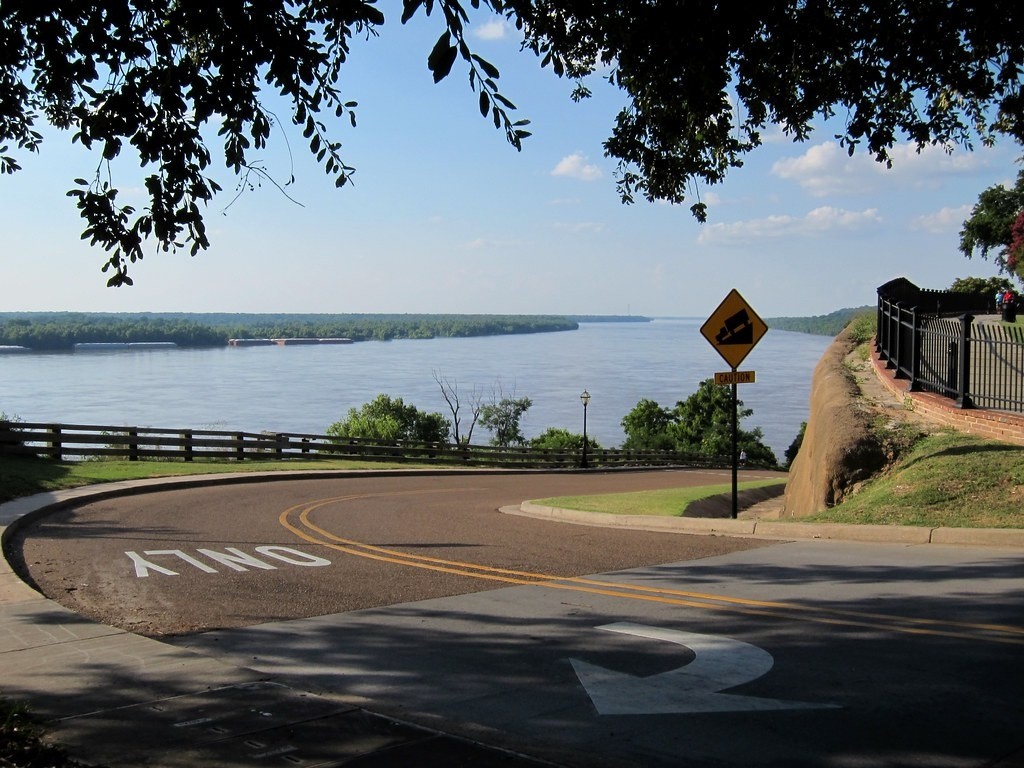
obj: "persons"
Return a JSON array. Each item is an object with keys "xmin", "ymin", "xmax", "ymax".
[{"xmin": 995, "ymin": 288, "xmax": 1012, "ymax": 315}]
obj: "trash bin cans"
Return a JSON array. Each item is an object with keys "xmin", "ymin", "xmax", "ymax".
[{"xmin": 1002, "ymin": 300, "xmax": 1016, "ymax": 323}]
